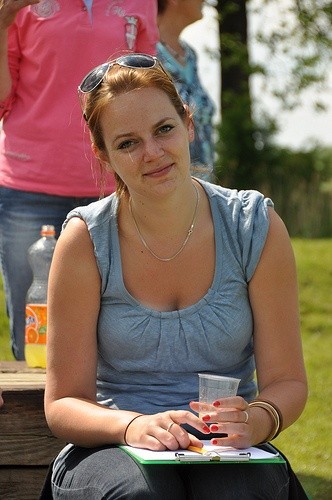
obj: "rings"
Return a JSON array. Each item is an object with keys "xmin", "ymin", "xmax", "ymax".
[
  {"xmin": 244, "ymin": 410, "xmax": 248, "ymax": 423},
  {"xmin": 167, "ymin": 422, "xmax": 174, "ymax": 431}
]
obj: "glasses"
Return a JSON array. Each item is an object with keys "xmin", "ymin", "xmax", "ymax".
[{"xmin": 77, "ymin": 54, "xmax": 173, "ymax": 111}]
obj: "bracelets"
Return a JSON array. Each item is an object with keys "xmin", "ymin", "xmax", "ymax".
[
  {"xmin": 249, "ymin": 399, "xmax": 283, "ymax": 446},
  {"xmin": 124, "ymin": 415, "xmax": 142, "ymax": 446}
]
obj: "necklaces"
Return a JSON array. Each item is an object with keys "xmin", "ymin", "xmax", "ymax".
[{"xmin": 128, "ymin": 182, "xmax": 200, "ymax": 262}]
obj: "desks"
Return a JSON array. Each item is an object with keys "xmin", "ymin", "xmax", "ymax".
[{"xmin": 0, "ymin": 361, "xmax": 76, "ymax": 500}]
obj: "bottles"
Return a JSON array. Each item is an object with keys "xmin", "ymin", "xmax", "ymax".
[{"xmin": 24, "ymin": 224, "xmax": 57, "ymax": 369}]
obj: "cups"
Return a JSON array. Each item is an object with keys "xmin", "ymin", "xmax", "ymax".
[{"xmin": 198, "ymin": 373, "xmax": 241, "ymax": 434}]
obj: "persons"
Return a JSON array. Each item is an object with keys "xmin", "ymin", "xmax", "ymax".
[
  {"xmin": 43, "ymin": 53, "xmax": 309, "ymax": 500},
  {"xmin": 155, "ymin": 0, "xmax": 216, "ymax": 185},
  {"xmin": 0, "ymin": 0, "xmax": 160, "ymax": 361}
]
